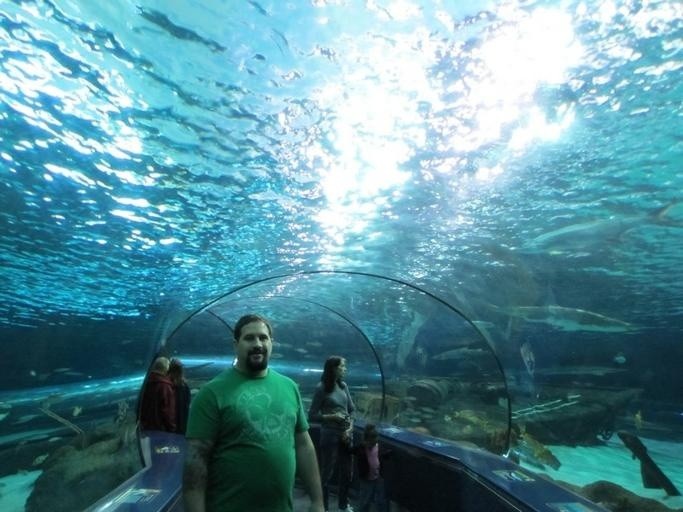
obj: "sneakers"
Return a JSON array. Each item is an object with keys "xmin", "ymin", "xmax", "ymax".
[{"xmin": 340, "ymin": 504, "xmax": 354, "ymax": 512}]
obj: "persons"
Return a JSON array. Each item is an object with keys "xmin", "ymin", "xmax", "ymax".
[
  {"xmin": 308, "ymin": 357, "xmax": 358, "ymax": 511},
  {"xmin": 166, "ymin": 357, "xmax": 190, "ymax": 437},
  {"xmin": 179, "ymin": 313, "xmax": 326, "ymax": 512},
  {"xmin": 139, "ymin": 354, "xmax": 177, "ymax": 435},
  {"xmin": 338, "ymin": 422, "xmax": 395, "ymax": 511}
]
